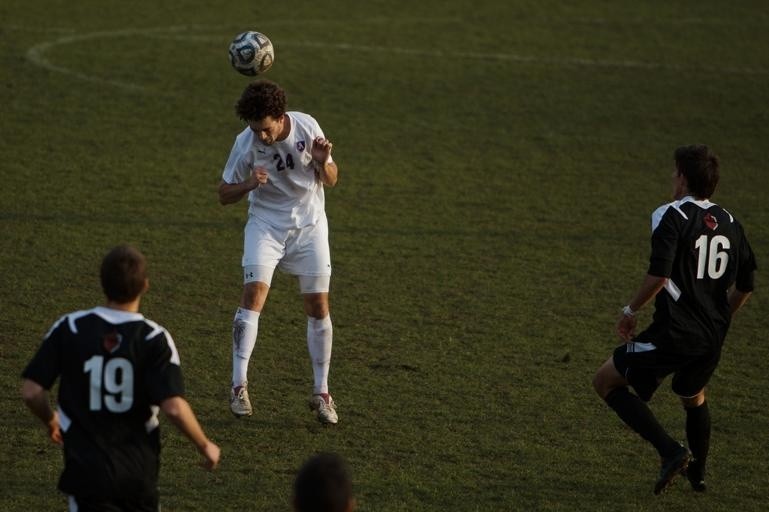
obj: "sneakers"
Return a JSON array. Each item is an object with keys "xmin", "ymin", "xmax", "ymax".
[
  {"xmin": 654, "ymin": 445, "xmax": 706, "ymax": 495},
  {"xmin": 310, "ymin": 395, "xmax": 338, "ymax": 424},
  {"xmin": 231, "ymin": 384, "xmax": 253, "ymax": 417}
]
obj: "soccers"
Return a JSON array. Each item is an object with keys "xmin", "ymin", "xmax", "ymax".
[{"xmin": 229, "ymin": 31, "xmax": 274, "ymax": 76}]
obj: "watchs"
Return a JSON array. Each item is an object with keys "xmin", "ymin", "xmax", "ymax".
[{"xmin": 622, "ymin": 304, "xmax": 640, "ymax": 319}]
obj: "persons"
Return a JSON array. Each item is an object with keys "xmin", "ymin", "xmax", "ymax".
[
  {"xmin": 291, "ymin": 453, "xmax": 354, "ymax": 511},
  {"xmin": 218, "ymin": 80, "xmax": 338, "ymax": 425},
  {"xmin": 19, "ymin": 243, "xmax": 220, "ymax": 512},
  {"xmin": 592, "ymin": 145, "xmax": 757, "ymax": 497}
]
